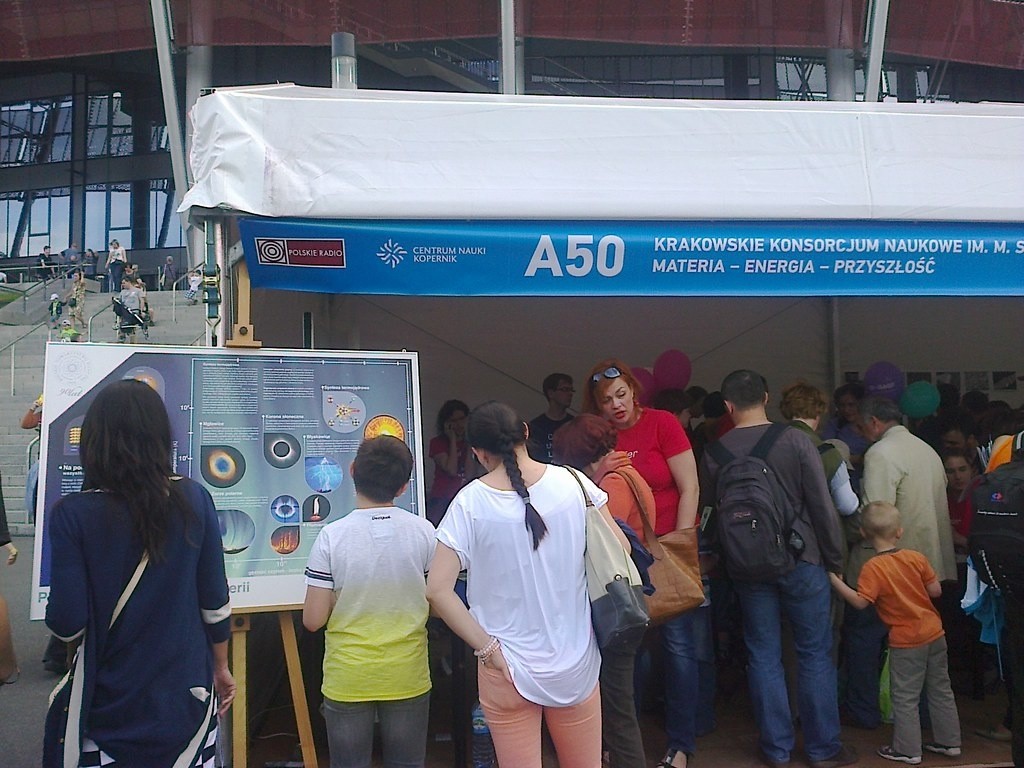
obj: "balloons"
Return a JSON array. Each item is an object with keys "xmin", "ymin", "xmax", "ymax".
[
  {"xmin": 630, "ymin": 349, "xmax": 691, "ymax": 406},
  {"xmin": 866, "ymin": 361, "xmax": 941, "ymax": 418}
]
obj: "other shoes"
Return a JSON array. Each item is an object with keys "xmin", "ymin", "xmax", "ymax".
[
  {"xmin": 42, "ymin": 651, "xmax": 68, "ymax": 674},
  {"xmin": 756, "ymin": 744, "xmax": 789, "ymax": 768},
  {"xmin": 713, "ymin": 667, "xmax": 748, "ymax": 695},
  {"xmin": 839, "ymin": 705, "xmax": 879, "ymax": 730},
  {"xmin": 976, "ymin": 722, "xmax": 1012, "ymax": 741},
  {"xmin": 809, "ymin": 745, "xmax": 858, "ymax": 768},
  {"xmin": 0, "ymin": 666, "xmax": 20, "ymax": 686},
  {"xmin": 694, "ymin": 713, "xmax": 720, "ymax": 737}
]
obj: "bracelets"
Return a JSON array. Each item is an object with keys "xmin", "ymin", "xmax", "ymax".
[
  {"xmin": 481, "ymin": 638, "xmax": 497, "ymax": 655},
  {"xmin": 480, "ymin": 643, "xmax": 500, "ymax": 665},
  {"xmin": 474, "ymin": 635, "xmax": 493, "ymax": 656},
  {"xmin": 4, "ymin": 665, "xmax": 20, "ymax": 684}
]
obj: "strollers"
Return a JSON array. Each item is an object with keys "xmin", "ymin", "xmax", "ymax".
[{"xmin": 112, "ymin": 297, "xmax": 149, "ymax": 341}]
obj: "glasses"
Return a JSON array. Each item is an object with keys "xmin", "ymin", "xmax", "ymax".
[
  {"xmin": 552, "ymin": 387, "xmax": 575, "ymax": 393},
  {"xmin": 590, "ymin": 367, "xmax": 625, "ymax": 388}
]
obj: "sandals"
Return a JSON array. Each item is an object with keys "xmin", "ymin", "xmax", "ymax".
[{"xmin": 656, "ymin": 746, "xmax": 689, "ymax": 768}]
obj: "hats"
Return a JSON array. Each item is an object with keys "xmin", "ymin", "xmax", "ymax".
[
  {"xmin": 62, "ymin": 320, "xmax": 70, "ymax": 326},
  {"xmin": 824, "ymin": 438, "xmax": 856, "ymax": 471},
  {"xmin": 50, "ymin": 293, "xmax": 58, "ymax": 300}
]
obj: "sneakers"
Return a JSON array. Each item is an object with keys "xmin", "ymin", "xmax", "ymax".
[
  {"xmin": 875, "ymin": 744, "xmax": 921, "ymax": 764},
  {"xmin": 925, "ymin": 741, "xmax": 961, "ymax": 756}
]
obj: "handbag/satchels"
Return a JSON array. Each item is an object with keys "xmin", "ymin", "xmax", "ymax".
[
  {"xmin": 68, "ymin": 298, "xmax": 76, "ymax": 307},
  {"xmin": 879, "ymin": 646, "xmax": 894, "ymax": 724},
  {"xmin": 596, "ymin": 469, "xmax": 706, "ymax": 628},
  {"xmin": 562, "ymin": 464, "xmax": 650, "ymax": 655},
  {"xmin": 160, "ymin": 264, "xmax": 166, "ymax": 286}
]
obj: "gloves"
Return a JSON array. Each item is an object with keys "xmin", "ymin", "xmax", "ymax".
[{"xmin": 30, "ymin": 400, "xmax": 39, "ymax": 412}]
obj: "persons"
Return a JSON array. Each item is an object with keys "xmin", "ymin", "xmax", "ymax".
[
  {"xmin": 424, "ymin": 400, "xmax": 631, "ymax": 768},
  {"xmin": 302, "ymin": 434, "xmax": 436, "ymax": 768},
  {"xmin": 38, "ymin": 239, "xmax": 202, "ymax": 342},
  {"xmin": 823, "ymin": 384, "xmax": 1024, "ymax": 768},
  {"xmin": 429, "ymin": 357, "xmax": 860, "ymax": 767},
  {"xmin": 44, "ymin": 378, "xmax": 237, "ymax": 768},
  {"xmin": 0, "ymin": 393, "xmax": 69, "ymax": 686}
]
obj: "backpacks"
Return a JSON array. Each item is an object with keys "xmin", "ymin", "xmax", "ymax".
[
  {"xmin": 706, "ymin": 420, "xmax": 805, "ymax": 595},
  {"xmin": 967, "ymin": 475, "xmax": 1024, "ymax": 597}
]
